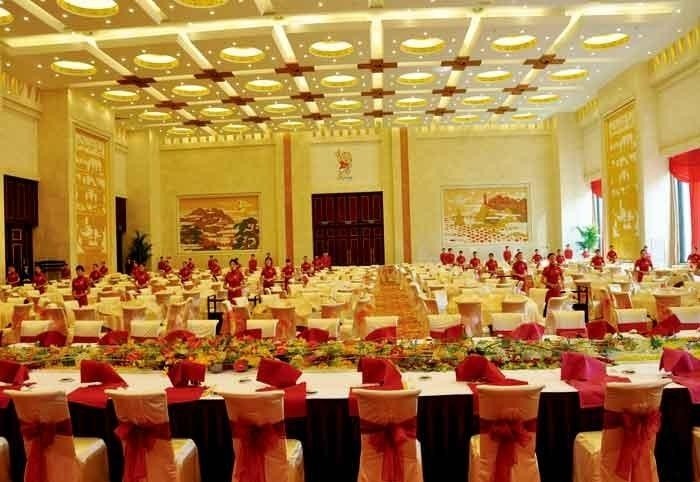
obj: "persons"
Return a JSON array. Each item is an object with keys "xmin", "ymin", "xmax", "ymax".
[
  {"xmin": 455, "ymin": 250, "xmax": 466, "ymax": 272},
  {"xmin": 6, "ymin": 266, "xmax": 20, "ymax": 286},
  {"xmin": 644, "ymin": 245, "xmax": 653, "ymax": 263},
  {"xmin": 556, "ymin": 249, "xmax": 564, "ymax": 264},
  {"xmin": 540, "ymin": 252, "xmax": 565, "ymax": 303},
  {"xmin": 503, "ymin": 246, "xmax": 512, "ymax": 264},
  {"xmin": 126, "ymin": 255, "xmax": 222, "ymax": 288},
  {"xmin": 582, "ymin": 247, "xmax": 590, "ymax": 258},
  {"xmin": 61, "ymin": 264, "xmax": 71, "ymax": 279},
  {"xmin": 469, "ymin": 251, "xmax": 481, "ymax": 277},
  {"xmin": 71, "ymin": 264, "xmax": 91, "ymax": 307},
  {"xmin": 687, "ymin": 247, "xmax": 700, "ymax": 271},
  {"xmin": 301, "ymin": 252, "xmax": 334, "ymax": 276},
  {"xmin": 223, "ymin": 258, "xmax": 247, "ymax": 305},
  {"xmin": 485, "ymin": 253, "xmax": 498, "ymax": 274},
  {"xmin": 100, "ymin": 262, "xmax": 109, "ymax": 276},
  {"xmin": 565, "ymin": 243, "xmax": 574, "ymax": 260},
  {"xmin": 439, "ymin": 248, "xmax": 446, "ymax": 264},
  {"xmin": 531, "ymin": 248, "xmax": 542, "ymax": 264},
  {"xmin": 588, "ymin": 248, "xmax": 606, "ymax": 273},
  {"xmin": 90, "ymin": 263, "xmax": 102, "ymax": 284},
  {"xmin": 260, "ymin": 256, "xmax": 278, "ymax": 295},
  {"xmin": 248, "ymin": 254, "xmax": 258, "ymax": 274},
  {"xmin": 511, "ymin": 252, "xmax": 528, "ymax": 290},
  {"xmin": 444, "ymin": 248, "xmax": 456, "ymax": 264},
  {"xmin": 282, "ymin": 258, "xmax": 296, "ymax": 292},
  {"xmin": 608, "ymin": 245, "xmax": 618, "ymax": 263},
  {"xmin": 32, "ymin": 265, "xmax": 48, "ymax": 294},
  {"xmin": 633, "ymin": 248, "xmax": 654, "ymax": 282}
]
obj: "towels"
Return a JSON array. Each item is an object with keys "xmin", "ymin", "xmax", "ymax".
[
  {"xmin": 97, "ymin": 330, "xmax": 128, "ymax": 345},
  {"xmin": 243, "ymin": 327, "xmax": 262, "ymax": 339},
  {"xmin": 660, "ymin": 347, "xmax": 699, "ymax": 374},
  {"xmin": 651, "ymin": 313, "xmax": 682, "ymax": 338},
  {"xmin": 0, "ymin": 386, "xmax": 23, "ymax": 407},
  {"xmin": 79, "ymin": 358, "xmax": 128, "ymax": 388},
  {"xmin": 667, "ymin": 370, "xmax": 700, "ymax": 405},
  {"xmin": 36, "ymin": 330, "xmax": 67, "ymax": 346},
  {"xmin": 515, "ymin": 322, "xmax": 545, "ymax": 339},
  {"xmin": 374, "ymin": 325, "xmax": 396, "ymax": 340},
  {"xmin": 566, "ymin": 374, "xmax": 633, "ymax": 409},
  {"xmin": 455, "ymin": 354, "xmax": 506, "ymax": 385},
  {"xmin": 1, "ymin": 360, "xmax": 31, "ymax": 384},
  {"xmin": 442, "ymin": 324, "xmax": 463, "ymax": 340},
  {"xmin": 256, "ymin": 357, "xmax": 303, "ymax": 388},
  {"xmin": 357, "ymin": 356, "xmax": 403, "ymax": 388},
  {"xmin": 168, "ymin": 361, "xmax": 206, "ymax": 386},
  {"xmin": 304, "ymin": 328, "xmax": 329, "ymax": 339},
  {"xmin": 165, "ymin": 329, "xmax": 196, "ymax": 342},
  {"xmin": 588, "ymin": 319, "xmax": 609, "ymax": 338},
  {"xmin": 163, "ymin": 383, "xmax": 211, "ymax": 408},
  {"xmin": 347, "ymin": 383, "xmax": 404, "ymax": 418},
  {"xmin": 65, "ymin": 386, "xmax": 113, "ymax": 410},
  {"xmin": 256, "ymin": 380, "xmax": 310, "ymax": 420},
  {"xmin": 468, "ymin": 379, "xmax": 531, "ymax": 417},
  {"xmin": 558, "ymin": 352, "xmax": 604, "ymax": 381}
]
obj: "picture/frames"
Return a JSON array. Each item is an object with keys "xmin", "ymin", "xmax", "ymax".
[
  {"xmin": 174, "ymin": 190, "xmax": 268, "ymax": 255},
  {"xmin": 437, "ymin": 180, "xmax": 536, "ymax": 251}
]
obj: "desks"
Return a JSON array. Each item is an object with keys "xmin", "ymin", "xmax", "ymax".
[{"xmin": 1, "ymin": 341, "xmax": 699, "ymax": 482}]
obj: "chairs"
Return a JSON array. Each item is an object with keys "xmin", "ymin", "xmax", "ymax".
[
  {"xmin": 104, "ymin": 387, "xmax": 201, "ymax": 481},
  {"xmin": 214, "ymin": 389, "xmax": 305, "ymax": 482},
  {"xmin": 246, "ymin": 319, "xmax": 279, "ymax": 338},
  {"xmin": 490, "ymin": 312, "xmax": 525, "ymax": 335},
  {"xmin": 614, "ymin": 307, "xmax": 648, "ymax": 336},
  {"xmin": 129, "ymin": 319, "xmax": 161, "ymax": 344},
  {"xmin": 363, "ymin": 315, "xmax": 399, "ymax": 340},
  {"xmin": 572, "ymin": 381, "xmax": 671, "ymax": 482},
  {"xmin": 308, "ymin": 319, "xmax": 339, "ymax": 340},
  {"xmin": 669, "ymin": 305, "xmax": 700, "ymax": 330},
  {"xmin": 551, "ymin": 310, "xmax": 586, "ymax": 337},
  {"xmin": 186, "ymin": 319, "xmax": 219, "ymax": 343},
  {"xmin": 426, "ymin": 313, "xmax": 464, "ymax": 338},
  {"xmin": 469, "ymin": 384, "xmax": 548, "ymax": 482},
  {"xmin": 73, "ymin": 319, "xmax": 104, "ymax": 344},
  {"xmin": 351, "ymin": 387, "xmax": 426, "ymax": 482},
  {"xmin": 19, "ymin": 319, "xmax": 50, "ymax": 345},
  {"xmin": 1, "ymin": 387, "xmax": 111, "ymax": 482}
]
